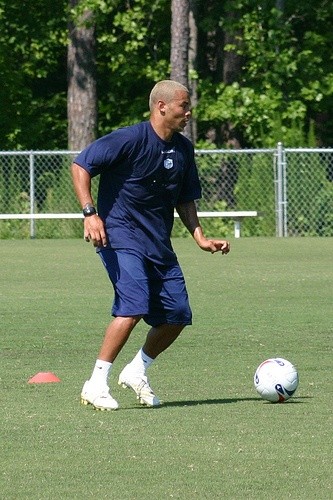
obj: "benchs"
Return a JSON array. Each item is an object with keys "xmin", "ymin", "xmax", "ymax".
[{"xmin": 0, "ymin": 211, "xmax": 258, "ymax": 240}]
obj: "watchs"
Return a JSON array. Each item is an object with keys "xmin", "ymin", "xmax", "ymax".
[{"xmin": 82, "ymin": 204, "xmax": 98, "ymax": 220}]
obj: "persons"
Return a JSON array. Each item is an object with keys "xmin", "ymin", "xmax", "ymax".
[{"xmin": 71, "ymin": 81, "xmax": 231, "ymax": 410}]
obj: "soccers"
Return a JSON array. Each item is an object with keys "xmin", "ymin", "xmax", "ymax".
[{"xmin": 253, "ymin": 358, "xmax": 298, "ymax": 402}]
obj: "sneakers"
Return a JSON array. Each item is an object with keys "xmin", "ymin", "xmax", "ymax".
[
  {"xmin": 80, "ymin": 380, "xmax": 119, "ymax": 411},
  {"xmin": 118, "ymin": 364, "xmax": 160, "ymax": 407}
]
